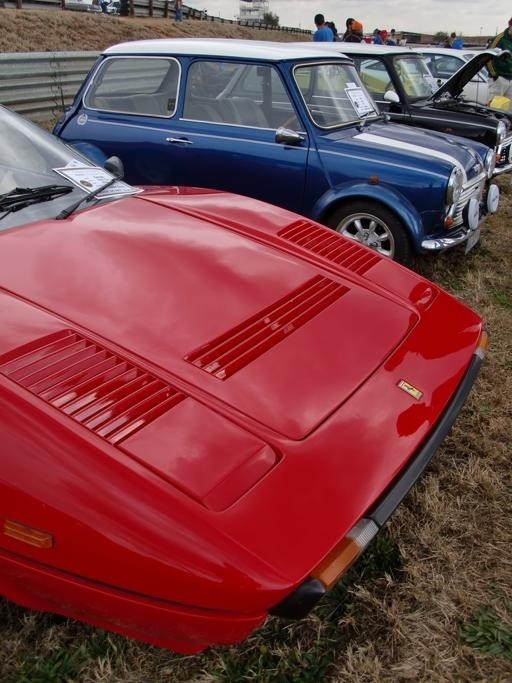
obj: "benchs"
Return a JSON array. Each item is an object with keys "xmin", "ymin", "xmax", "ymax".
[{"xmin": 96, "ymin": 94, "xmax": 175, "ymax": 118}]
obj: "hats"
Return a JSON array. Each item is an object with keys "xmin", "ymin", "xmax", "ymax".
[{"xmin": 352, "ymin": 20, "xmax": 363, "ymax": 31}]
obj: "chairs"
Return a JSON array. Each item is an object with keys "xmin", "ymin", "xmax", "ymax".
[
  {"xmin": 188, "ymin": 102, "xmax": 220, "ymax": 123},
  {"xmin": 220, "ymin": 94, "xmax": 269, "ymax": 127}
]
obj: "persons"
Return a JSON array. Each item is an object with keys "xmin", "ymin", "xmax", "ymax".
[
  {"xmin": 313, "ymin": 13, "xmax": 335, "ymax": 42},
  {"xmin": 443, "ymin": 35, "xmax": 452, "ymax": 48},
  {"xmin": 173, "ymin": 0, "xmax": 183, "ymax": 22},
  {"xmin": 450, "ymin": 32, "xmax": 464, "ymax": 49},
  {"xmin": 486, "ymin": 16, "xmax": 511, "ymax": 113},
  {"xmin": 344, "ymin": 20, "xmax": 364, "ymax": 43},
  {"xmin": 325, "ymin": 20, "xmax": 341, "ymax": 42},
  {"xmin": 361, "ymin": 27, "xmax": 408, "ymax": 45},
  {"xmin": 341, "ymin": 17, "xmax": 355, "ymax": 40}
]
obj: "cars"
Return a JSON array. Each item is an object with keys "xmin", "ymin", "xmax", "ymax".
[
  {"xmin": 65, "ymin": 0, "xmax": 119, "ymax": 15},
  {"xmin": 214, "ymin": 46, "xmax": 511, "ymax": 181},
  {"xmin": 54, "ymin": 38, "xmax": 500, "ymax": 269},
  {"xmin": 1, "ymin": 101, "xmax": 490, "ymax": 653}
]
